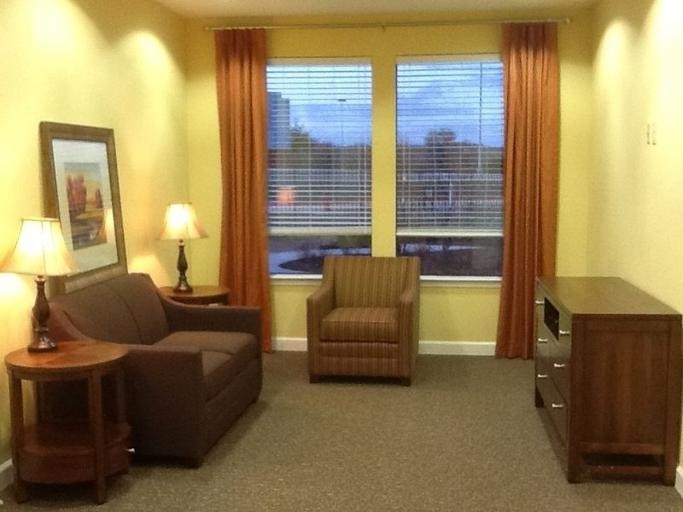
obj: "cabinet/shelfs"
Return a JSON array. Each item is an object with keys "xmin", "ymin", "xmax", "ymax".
[{"xmin": 533, "ymin": 275, "xmax": 683, "ymax": 486}]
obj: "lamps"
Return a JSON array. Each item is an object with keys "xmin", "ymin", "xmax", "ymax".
[
  {"xmin": 158, "ymin": 203, "xmax": 209, "ymax": 292},
  {"xmin": 0, "ymin": 217, "xmax": 82, "ymax": 352}
]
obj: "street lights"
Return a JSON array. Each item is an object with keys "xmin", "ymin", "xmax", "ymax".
[{"xmin": 337, "ymin": 97, "xmax": 349, "ymax": 181}]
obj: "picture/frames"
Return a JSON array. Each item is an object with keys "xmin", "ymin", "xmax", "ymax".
[{"xmin": 39, "ymin": 121, "xmax": 129, "ymax": 293}]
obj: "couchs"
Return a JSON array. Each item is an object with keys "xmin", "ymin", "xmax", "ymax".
[
  {"xmin": 306, "ymin": 255, "xmax": 420, "ymax": 385},
  {"xmin": 33, "ymin": 273, "xmax": 263, "ymax": 467}
]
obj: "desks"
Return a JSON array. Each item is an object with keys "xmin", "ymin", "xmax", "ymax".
[
  {"xmin": 4, "ymin": 340, "xmax": 131, "ymax": 505},
  {"xmin": 158, "ymin": 287, "xmax": 230, "ymax": 306}
]
